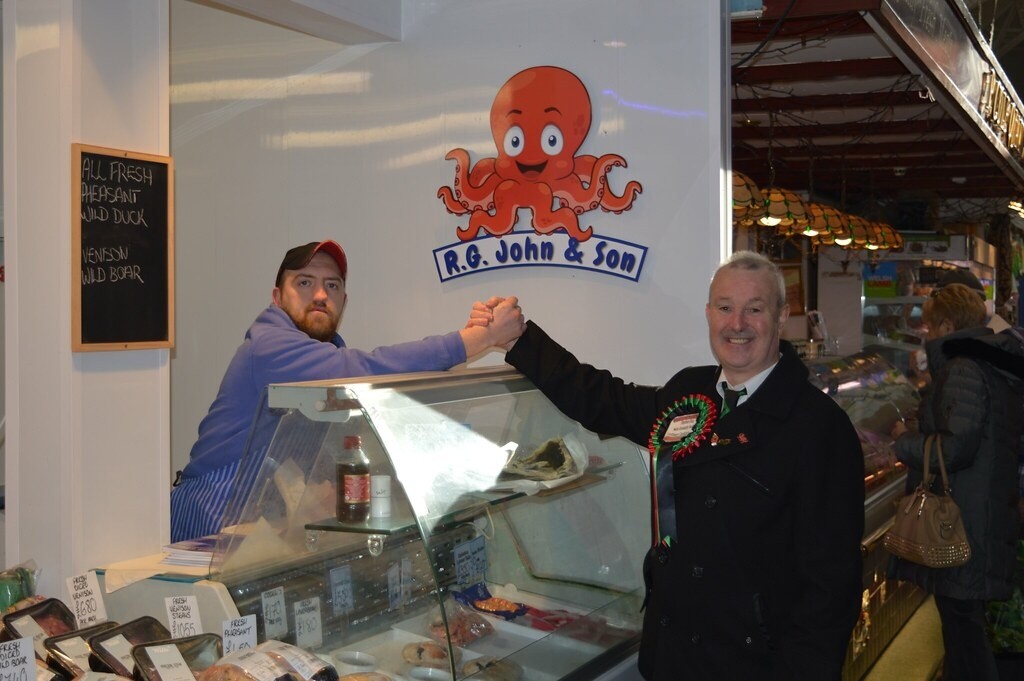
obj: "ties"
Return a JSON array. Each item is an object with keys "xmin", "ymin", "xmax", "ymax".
[{"xmin": 717, "ymin": 382, "xmax": 747, "ymax": 420}]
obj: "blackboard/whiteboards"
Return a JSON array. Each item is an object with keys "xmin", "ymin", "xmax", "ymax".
[{"xmin": 69, "ymin": 139, "xmax": 179, "ymax": 354}]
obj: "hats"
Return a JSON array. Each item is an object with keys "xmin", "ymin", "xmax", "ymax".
[
  {"xmin": 931, "ymin": 268, "xmax": 986, "ymax": 301},
  {"xmin": 275, "ymin": 240, "xmax": 348, "ymax": 288}
]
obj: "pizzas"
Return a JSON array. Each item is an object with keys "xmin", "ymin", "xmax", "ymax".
[{"xmin": 501, "ymin": 437, "xmax": 572, "ymax": 480}]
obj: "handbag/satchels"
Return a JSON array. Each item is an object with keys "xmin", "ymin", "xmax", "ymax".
[{"xmin": 884, "ymin": 433, "xmax": 972, "ymax": 568}]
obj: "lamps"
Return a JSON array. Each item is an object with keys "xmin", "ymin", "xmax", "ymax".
[{"xmin": 730, "ymin": 108, "xmax": 904, "ymax": 249}]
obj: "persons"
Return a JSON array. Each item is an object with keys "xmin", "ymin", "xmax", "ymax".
[
  {"xmin": 170, "ymin": 241, "xmax": 524, "ymax": 541},
  {"xmin": 466, "ymin": 251, "xmax": 864, "ymax": 681},
  {"xmin": 885, "ymin": 268, "xmax": 1024, "ymax": 681}
]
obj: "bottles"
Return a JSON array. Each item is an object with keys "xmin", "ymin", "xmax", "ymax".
[{"xmin": 336, "ymin": 436, "xmax": 371, "ymax": 526}]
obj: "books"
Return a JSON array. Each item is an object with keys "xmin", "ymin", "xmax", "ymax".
[{"xmin": 160, "ymin": 533, "xmax": 246, "ymax": 568}]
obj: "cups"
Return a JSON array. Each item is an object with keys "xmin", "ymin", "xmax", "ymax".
[
  {"xmin": 370, "ymin": 476, "xmax": 391, "ymax": 518},
  {"xmin": 334, "ymin": 651, "xmax": 377, "ymax": 676},
  {"xmin": 408, "ymin": 668, "xmax": 451, "ymax": 681}
]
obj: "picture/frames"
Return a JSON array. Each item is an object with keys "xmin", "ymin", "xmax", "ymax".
[{"xmin": 778, "ymin": 264, "xmax": 806, "ymax": 316}]
